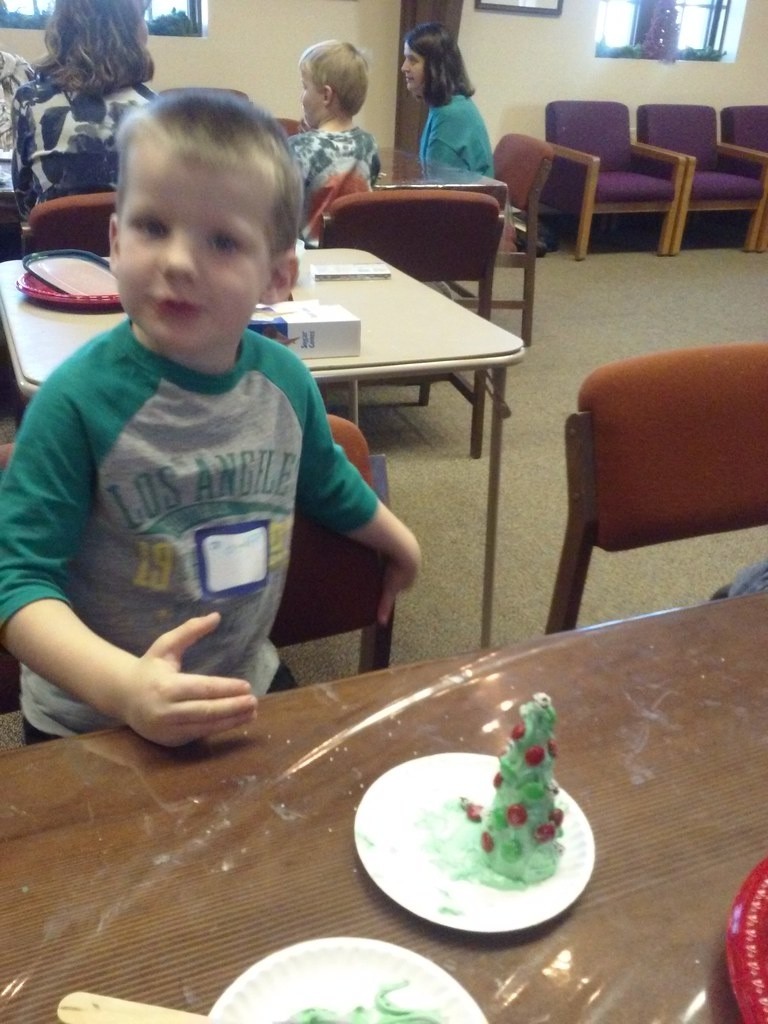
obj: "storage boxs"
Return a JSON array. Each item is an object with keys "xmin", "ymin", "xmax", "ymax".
[{"xmin": 243, "ymin": 300, "xmax": 361, "ymax": 357}]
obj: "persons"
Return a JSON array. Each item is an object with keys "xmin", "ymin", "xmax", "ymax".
[
  {"xmin": 400, "ymin": 22, "xmax": 493, "ymax": 178},
  {"xmin": 0, "ymin": 91, "xmax": 423, "ymax": 747},
  {"xmin": 0, "ymin": 50, "xmax": 40, "ymax": 192},
  {"xmin": 11, "ymin": 0, "xmax": 162, "ymax": 223},
  {"xmin": 283, "ymin": 39, "xmax": 381, "ymax": 249}
]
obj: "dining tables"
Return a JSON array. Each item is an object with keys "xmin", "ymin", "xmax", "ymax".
[{"xmin": 0, "ymin": 591, "xmax": 768, "ymax": 1024}]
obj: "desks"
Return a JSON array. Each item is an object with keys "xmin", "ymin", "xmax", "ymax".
[{"xmin": 0, "ymin": 247, "xmax": 527, "ymax": 648}]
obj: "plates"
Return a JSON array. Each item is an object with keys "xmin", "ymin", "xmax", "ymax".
[
  {"xmin": 723, "ymin": 856, "xmax": 767, "ymax": 1024},
  {"xmin": 353, "ymin": 751, "xmax": 597, "ymax": 934},
  {"xmin": 206, "ymin": 934, "xmax": 491, "ymax": 1023},
  {"xmin": 15, "ymin": 271, "xmax": 123, "ymax": 309}
]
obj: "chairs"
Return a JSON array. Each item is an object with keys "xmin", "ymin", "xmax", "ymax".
[
  {"xmin": 544, "ymin": 342, "xmax": 768, "ymax": 635},
  {"xmin": 320, "ymin": 189, "xmax": 505, "ymax": 461},
  {"xmin": 540, "ymin": 101, "xmax": 768, "ymax": 261},
  {"xmin": 21, "ymin": 190, "xmax": 117, "ymax": 255},
  {"xmin": 491, "ymin": 133, "xmax": 553, "ymax": 347}
]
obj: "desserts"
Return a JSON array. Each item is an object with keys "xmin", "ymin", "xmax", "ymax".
[{"xmin": 466, "ymin": 691, "xmax": 564, "ymax": 889}]
